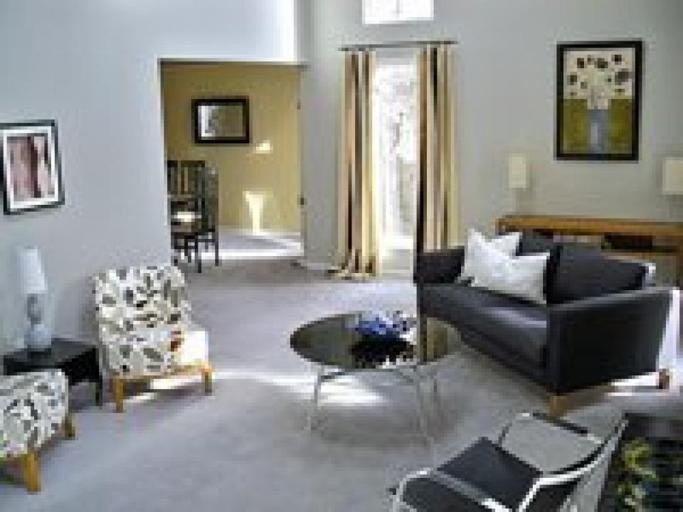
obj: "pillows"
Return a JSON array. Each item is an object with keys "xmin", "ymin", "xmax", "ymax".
[{"xmin": 456, "ymin": 230, "xmax": 647, "ymax": 306}]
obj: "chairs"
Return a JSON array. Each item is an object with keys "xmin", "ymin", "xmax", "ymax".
[
  {"xmin": 90, "ymin": 260, "xmax": 217, "ymax": 413},
  {"xmin": 166, "ymin": 158, "xmax": 223, "ymax": 274},
  {"xmin": 385, "ymin": 412, "xmax": 629, "ymax": 512},
  {"xmin": 0, "ymin": 365, "xmax": 76, "ymax": 494}
]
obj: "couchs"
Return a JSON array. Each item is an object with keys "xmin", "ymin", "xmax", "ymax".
[{"xmin": 414, "ymin": 234, "xmax": 681, "ymax": 413}]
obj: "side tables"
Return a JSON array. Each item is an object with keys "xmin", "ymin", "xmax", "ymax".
[{"xmin": 4, "ymin": 336, "xmax": 107, "ymax": 408}]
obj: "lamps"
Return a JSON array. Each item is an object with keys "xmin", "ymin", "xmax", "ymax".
[{"xmin": 18, "ymin": 245, "xmax": 52, "ymax": 352}]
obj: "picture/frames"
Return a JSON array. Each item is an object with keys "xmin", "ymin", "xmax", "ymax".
[
  {"xmin": 554, "ymin": 38, "xmax": 644, "ymax": 163},
  {"xmin": 191, "ymin": 96, "xmax": 252, "ymax": 144},
  {"xmin": 0, "ymin": 120, "xmax": 63, "ymax": 215}
]
